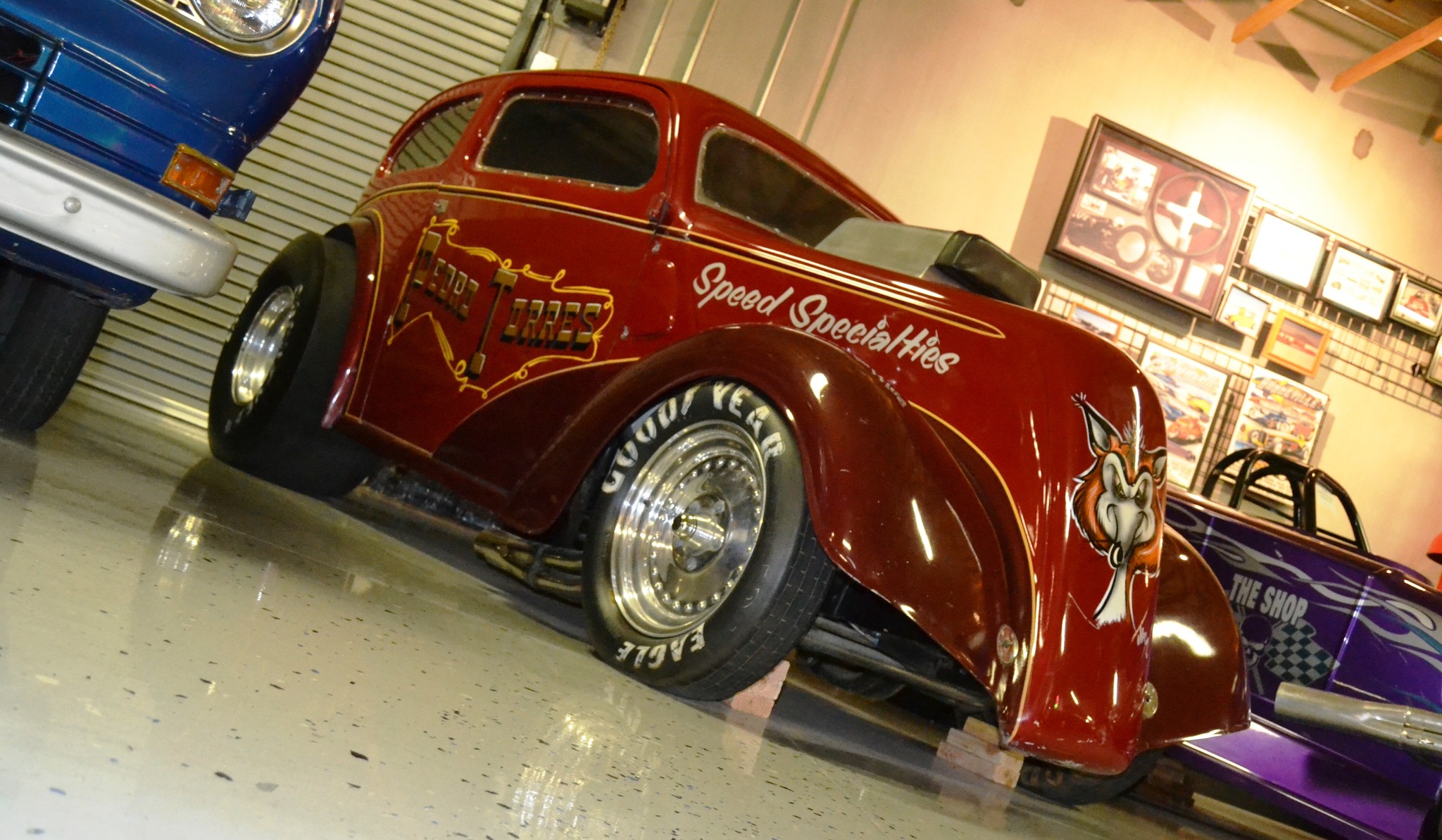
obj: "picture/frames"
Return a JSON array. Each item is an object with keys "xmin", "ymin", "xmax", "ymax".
[
  {"xmin": 1223, "ymin": 364, "xmax": 1330, "ymax": 499},
  {"xmin": 1215, "ymin": 284, "xmax": 1271, "ymax": 343},
  {"xmin": 1240, "ymin": 206, "xmax": 1330, "ymax": 296},
  {"xmin": 1044, "ymin": 114, "xmax": 1258, "ymax": 324},
  {"xmin": 1388, "ymin": 272, "xmax": 1442, "ymax": 337},
  {"xmin": 1136, "ymin": 335, "xmax": 1233, "ymax": 493},
  {"xmin": 1260, "ymin": 308, "xmax": 1333, "ymax": 379},
  {"xmin": 1065, "ymin": 301, "xmax": 1124, "ymax": 342},
  {"xmin": 1424, "ymin": 334, "xmax": 1442, "ymax": 387},
  {"xmin": 1314, "ymin": 239, "xmax": 1401, "ymax": 326}
]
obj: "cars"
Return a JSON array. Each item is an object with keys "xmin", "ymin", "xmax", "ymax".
[
  {"xmin": 1, "ymin": 0, "xmax": 343, "ymax": 433},
  {"xmin": 1150, "ymin": 453, "xmax": 1442, "ymax": 840},
  {"xmin": 212, "ymin": 74, "xmax": 1257, "ymax": 808}
]
